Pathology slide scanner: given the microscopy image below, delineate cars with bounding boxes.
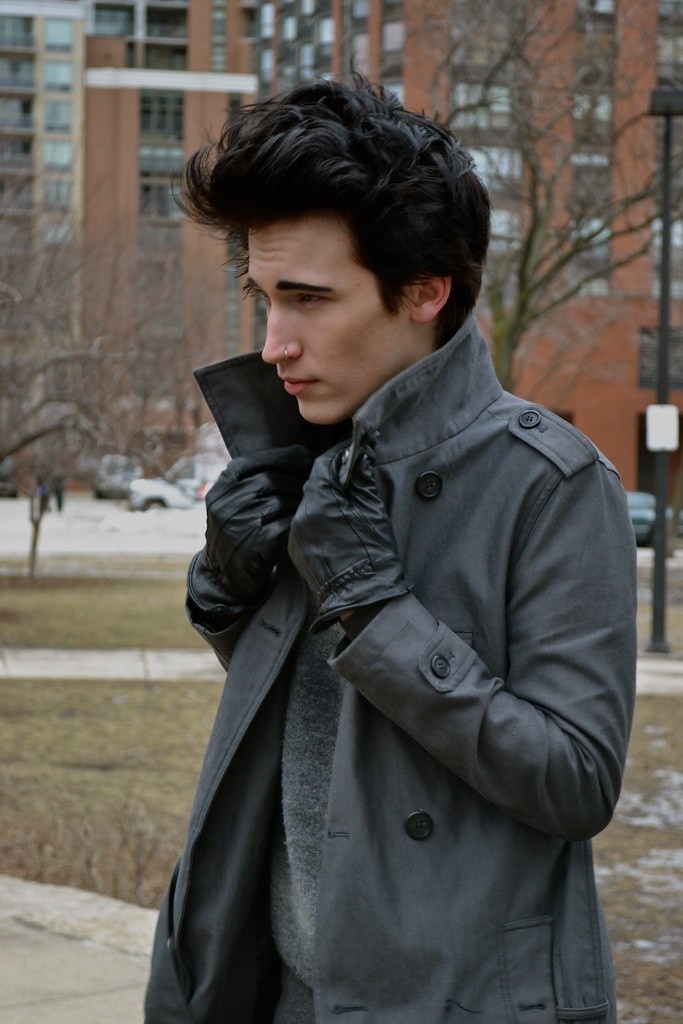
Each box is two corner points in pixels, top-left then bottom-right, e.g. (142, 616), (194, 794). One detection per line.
(623, 491), (673, 545)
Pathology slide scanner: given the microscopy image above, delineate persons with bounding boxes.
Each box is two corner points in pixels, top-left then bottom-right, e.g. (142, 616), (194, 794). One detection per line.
(35, 474), (64, 512)
(142, 54), (638, 1024)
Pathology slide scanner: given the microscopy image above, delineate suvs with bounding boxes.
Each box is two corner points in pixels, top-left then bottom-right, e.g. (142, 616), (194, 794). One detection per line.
(130, 455), (198, 514)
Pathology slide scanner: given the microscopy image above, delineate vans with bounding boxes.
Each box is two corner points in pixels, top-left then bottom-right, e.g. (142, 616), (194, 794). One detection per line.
(95, 455), (144, 499)
(0, 454), (20, 497)
(195, 422), (234, 505)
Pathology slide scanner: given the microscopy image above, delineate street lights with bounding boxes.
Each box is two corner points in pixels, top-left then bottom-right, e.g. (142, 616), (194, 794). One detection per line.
(645, 76), (683, 652)
